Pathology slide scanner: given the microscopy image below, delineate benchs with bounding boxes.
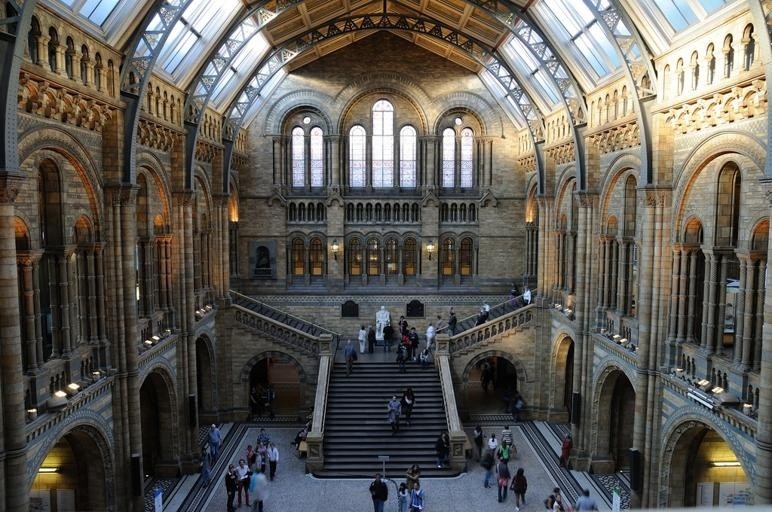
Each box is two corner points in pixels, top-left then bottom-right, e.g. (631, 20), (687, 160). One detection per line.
(463, 431), (473, 459)
(299, 440), (309, 457)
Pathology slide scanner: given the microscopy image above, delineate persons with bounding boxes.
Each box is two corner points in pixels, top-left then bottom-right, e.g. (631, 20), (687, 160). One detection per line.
(435, 431), (450, 469)
(388, 395), (400, 437)
(398, 464), (424, 512)
(249, 387), (262, 419)
(207, 424), (222, 461)
(473, 426), (527, 511)
(478, 360), (524, 422)
(343, 306), (457, 375)
(475, 302), (490, 342)
(400, 387), (415, 426)
(224, 428), (279, 512)
(290, 406), (313, 448)
(369, 474), (388, 512)
(200, 448), (212, 488)
(510, 282), (531, 310)
(544, 487), (597, 512)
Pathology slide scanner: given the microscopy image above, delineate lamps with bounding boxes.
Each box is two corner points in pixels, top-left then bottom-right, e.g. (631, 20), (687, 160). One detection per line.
(707, 461), (741, 467)
(38, 466), (64, 474)
(331, 238), (339, 260)
(554, 303), (753, 417)
(27, 304), (213, 421)
(426, 240), (435, 261)
